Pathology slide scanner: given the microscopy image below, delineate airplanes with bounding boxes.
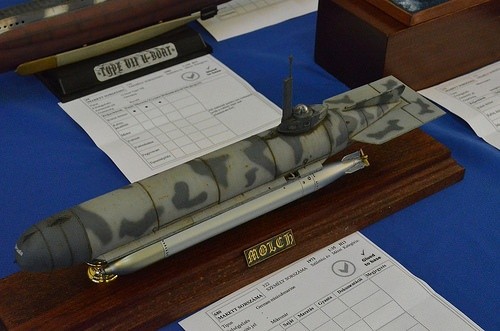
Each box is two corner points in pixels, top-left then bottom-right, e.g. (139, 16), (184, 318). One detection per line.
(0, 0), (232, 76)
(12, 54), (447, 285)
